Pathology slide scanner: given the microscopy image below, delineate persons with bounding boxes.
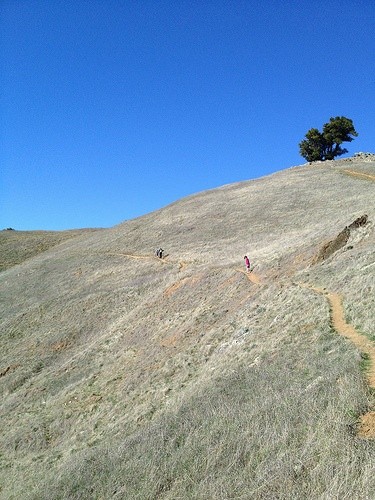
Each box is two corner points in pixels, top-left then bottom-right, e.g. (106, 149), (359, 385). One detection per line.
(156, 250), (159, 257)
(244, 255), (251, 273)
(159, 248), (164, 258)
